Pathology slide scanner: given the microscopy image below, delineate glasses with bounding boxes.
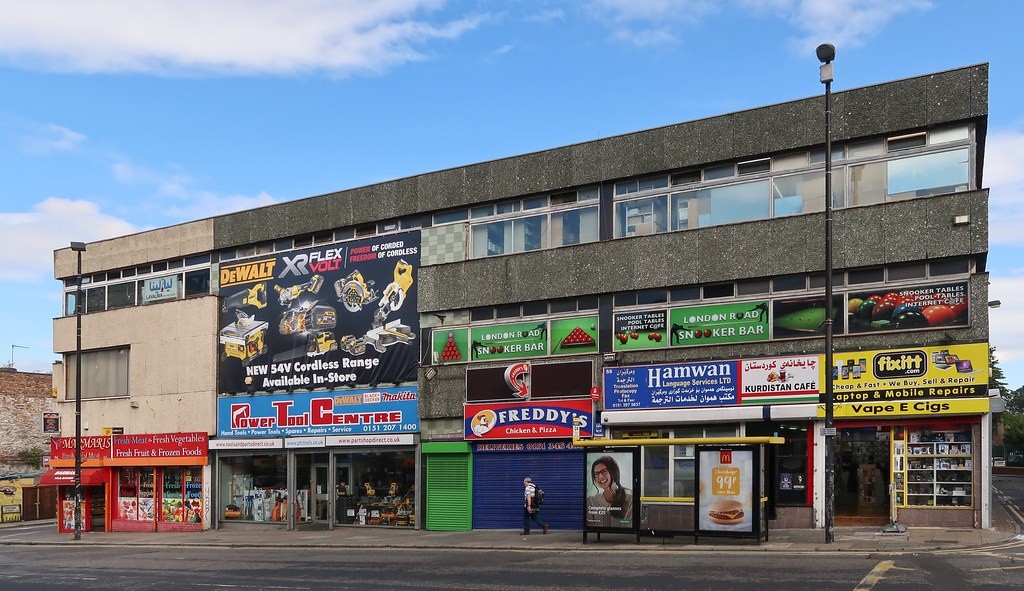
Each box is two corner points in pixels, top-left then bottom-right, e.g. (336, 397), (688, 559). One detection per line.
(593, 468), (609, 478)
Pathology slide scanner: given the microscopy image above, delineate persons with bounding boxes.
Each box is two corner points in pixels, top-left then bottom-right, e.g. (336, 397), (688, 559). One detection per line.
(864, 455), (881, 469)
(844, 457), (859, 510)
(587, 456), (633, 528)
(336, 478), (347, 499)
(520, 477), (549, 535)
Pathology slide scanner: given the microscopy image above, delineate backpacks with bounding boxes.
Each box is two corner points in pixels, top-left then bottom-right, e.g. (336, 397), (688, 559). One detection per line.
(526, 485), (545, 508)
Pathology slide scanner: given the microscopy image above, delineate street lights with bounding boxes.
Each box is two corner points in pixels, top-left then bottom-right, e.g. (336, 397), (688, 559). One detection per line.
(816, 42), (835, 543)
(70, 241), (86, 540)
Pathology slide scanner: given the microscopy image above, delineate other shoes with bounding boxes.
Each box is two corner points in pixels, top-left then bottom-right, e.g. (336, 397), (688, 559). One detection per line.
(520, 531), (530, 535)
(543, 524), (548, 534)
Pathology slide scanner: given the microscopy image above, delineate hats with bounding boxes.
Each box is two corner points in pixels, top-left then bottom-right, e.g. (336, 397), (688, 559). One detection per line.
(524, 478), (533, 483)
